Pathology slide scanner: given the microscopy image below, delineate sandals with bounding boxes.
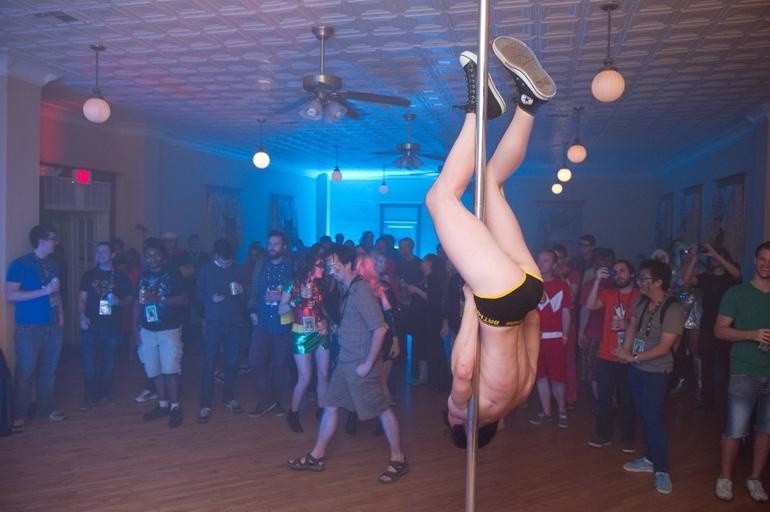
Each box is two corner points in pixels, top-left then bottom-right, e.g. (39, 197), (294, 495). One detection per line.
(567, 400), (577, 411)
(287, 452), (325, 471)
(378, 458), (409, 484)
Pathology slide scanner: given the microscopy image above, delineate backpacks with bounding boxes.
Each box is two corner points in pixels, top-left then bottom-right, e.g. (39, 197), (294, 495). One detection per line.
(658, 296), (693, 395)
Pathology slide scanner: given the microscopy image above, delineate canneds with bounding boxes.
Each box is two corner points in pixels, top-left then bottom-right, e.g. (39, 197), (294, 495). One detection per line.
(52, 277), (60, 283)
(107, 293), (114, 307)
(139, 289), (146, 303)
(230, 281), (238, 296)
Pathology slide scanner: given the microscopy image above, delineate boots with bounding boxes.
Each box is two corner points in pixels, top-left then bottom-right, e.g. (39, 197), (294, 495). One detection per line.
(409, 360), (430, 387)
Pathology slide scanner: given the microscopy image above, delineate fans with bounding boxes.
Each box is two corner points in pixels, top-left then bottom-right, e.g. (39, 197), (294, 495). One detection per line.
(369, 111), (446, 171)
(258, 25), (411, 124)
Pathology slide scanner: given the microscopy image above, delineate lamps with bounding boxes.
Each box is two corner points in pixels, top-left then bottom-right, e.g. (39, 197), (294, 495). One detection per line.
(81, 44), (112, 124)
(393, 153), (423, 171)
(550, 106), (589, 195)
(296, 91), (348, 123)
(251, 118), (387, 195)
(590, 1), (626, 103)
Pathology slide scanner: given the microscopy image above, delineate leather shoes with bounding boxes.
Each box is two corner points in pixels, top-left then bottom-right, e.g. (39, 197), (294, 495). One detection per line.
(8, 416), (25, 433)
(49, 410), (65, 421)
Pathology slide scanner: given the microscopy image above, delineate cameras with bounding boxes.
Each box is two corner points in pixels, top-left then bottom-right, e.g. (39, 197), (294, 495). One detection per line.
(697, 245), (708, 253)
(680, 248), (693, 256)
(607, 267), (616, 277)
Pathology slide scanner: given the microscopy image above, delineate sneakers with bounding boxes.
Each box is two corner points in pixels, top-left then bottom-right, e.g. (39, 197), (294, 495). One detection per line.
(622, 440), (636, 453)
(715, 478), (734, 501)
(458, 51), (507, 121)
(100, 396), (116, 405)
(133, 390), (401, 435)
(655, 471), (673, 494)
(558, 412), (570, 428)
(587, 437), (611, 447)
(746, 478), (768, 502)
(528, 412), (552, 425)
(490, 35), (558, 105)
(622, 456), (654, 473)
(79, 400), (97, 412)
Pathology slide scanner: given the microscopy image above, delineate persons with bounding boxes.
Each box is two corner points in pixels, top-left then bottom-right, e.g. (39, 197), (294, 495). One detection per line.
(424, 34), (557, 451)
(3, 223), (66, 433)
